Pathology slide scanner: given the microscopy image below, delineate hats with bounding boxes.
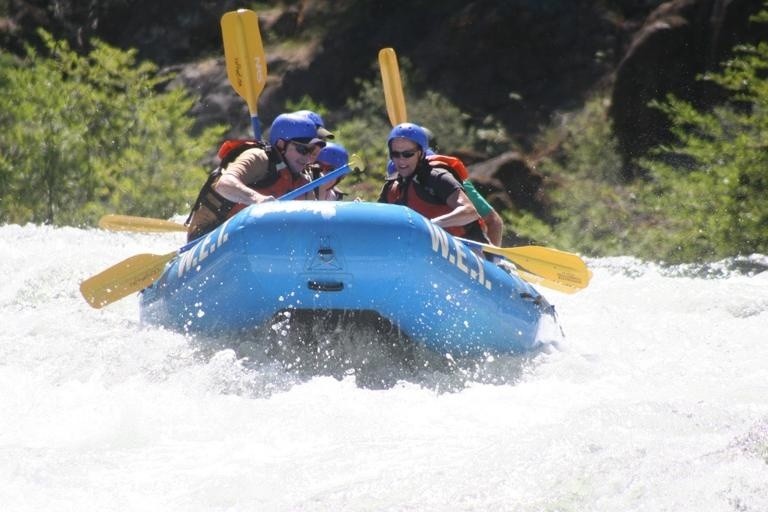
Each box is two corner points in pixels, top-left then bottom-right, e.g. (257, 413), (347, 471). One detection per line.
(290, 138), (326, 148)
(316, 126), (334, 139)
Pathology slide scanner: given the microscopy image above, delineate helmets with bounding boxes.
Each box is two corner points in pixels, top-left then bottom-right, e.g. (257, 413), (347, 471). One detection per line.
(293, 110), (323, 126)
(420, 127), (437, 152)
(388, 123), (426, 153)
(270, 114), (316, 145)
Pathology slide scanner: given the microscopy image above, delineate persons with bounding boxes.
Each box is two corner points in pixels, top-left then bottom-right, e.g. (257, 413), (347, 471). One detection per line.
(183, 111), (337, 247)
(315, 142), (350, 200)
(352, 120), (493, 263)
(276, 110), (334, 199)
(419, 127), (503, 259)
(388, 158), (397, 175)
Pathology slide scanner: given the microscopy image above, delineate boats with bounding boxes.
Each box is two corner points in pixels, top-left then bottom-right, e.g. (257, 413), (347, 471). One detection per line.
(125, 194), (553, 363)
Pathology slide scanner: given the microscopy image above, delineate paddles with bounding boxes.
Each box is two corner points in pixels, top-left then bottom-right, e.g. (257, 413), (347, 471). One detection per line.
(219, 7), (268, 143)
(379, 47), (407, 127)
(450, 235), (593, 295)
(79, 156), (365, 311)
(98, 212), (193, 235)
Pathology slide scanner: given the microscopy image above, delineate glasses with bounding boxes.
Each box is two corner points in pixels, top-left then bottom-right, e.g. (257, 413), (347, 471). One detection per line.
(391, 151), (420, 158)
(293, 143), (318, 155)
(314, 162), (334, 173)
